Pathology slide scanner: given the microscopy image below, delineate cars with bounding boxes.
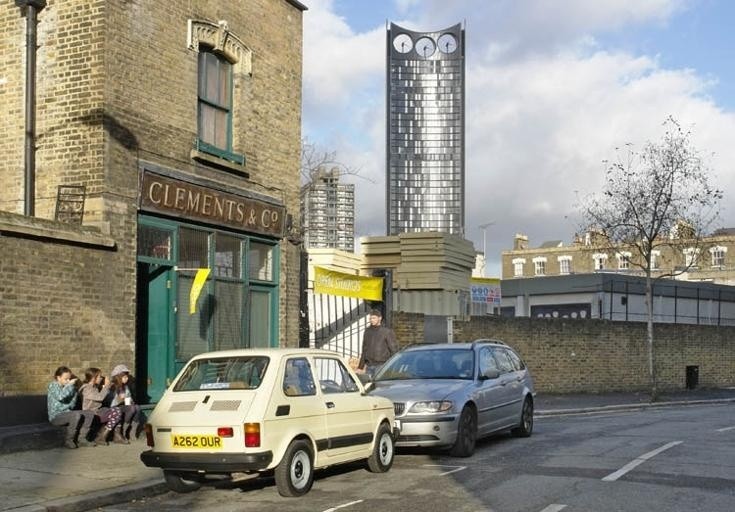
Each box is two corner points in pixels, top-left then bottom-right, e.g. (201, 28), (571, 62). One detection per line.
(356, 336), (538, 462)
(133, 346), (400, 500)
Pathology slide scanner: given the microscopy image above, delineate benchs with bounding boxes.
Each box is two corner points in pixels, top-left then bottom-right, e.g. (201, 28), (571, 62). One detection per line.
(0, 394), (106, 456)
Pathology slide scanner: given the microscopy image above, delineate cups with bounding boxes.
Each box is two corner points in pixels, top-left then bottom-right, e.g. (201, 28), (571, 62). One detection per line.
(123, 397), (131, 405)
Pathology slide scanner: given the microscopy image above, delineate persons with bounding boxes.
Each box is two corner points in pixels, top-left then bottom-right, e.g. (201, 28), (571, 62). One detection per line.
(47, 366), (97, 450)
(357, 310), (398, 382)
(105, 365), (141, 441)
(78, 368), (129, 446)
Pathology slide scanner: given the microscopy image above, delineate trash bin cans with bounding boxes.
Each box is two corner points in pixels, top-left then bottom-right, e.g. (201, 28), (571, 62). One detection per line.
(686, 366), (699, 390)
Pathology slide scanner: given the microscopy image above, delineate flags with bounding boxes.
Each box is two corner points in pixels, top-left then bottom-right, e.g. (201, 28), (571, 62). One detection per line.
(190, 268), (211, 313)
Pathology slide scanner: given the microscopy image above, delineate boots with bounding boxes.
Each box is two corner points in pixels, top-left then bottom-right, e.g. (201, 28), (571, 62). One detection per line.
(113, 424), (127, 444)
(65, 427), (77, 448)
(130, 421), (140, 441)
(78, 426), (96, 447)
(96, 426), (111, 446)
(122, 421), (130, 442)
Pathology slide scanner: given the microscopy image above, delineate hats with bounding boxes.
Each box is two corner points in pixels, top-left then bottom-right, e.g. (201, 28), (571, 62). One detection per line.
(111, 365), (130, 377)
